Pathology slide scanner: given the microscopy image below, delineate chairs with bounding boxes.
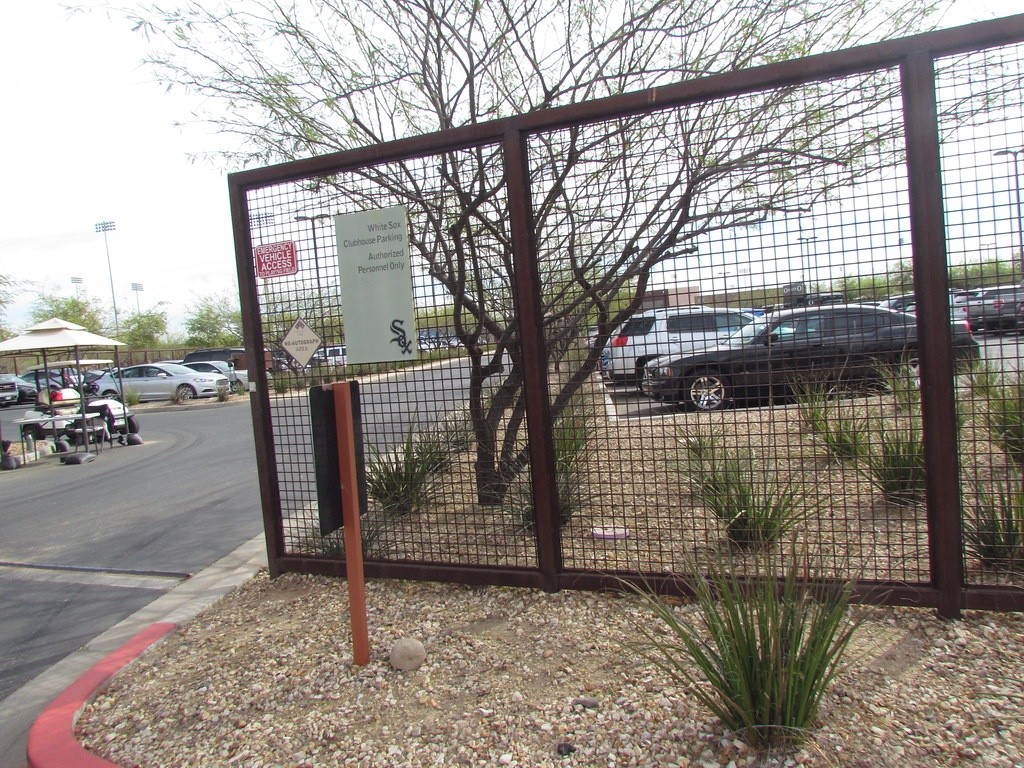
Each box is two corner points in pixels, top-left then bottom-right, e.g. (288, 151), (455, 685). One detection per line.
(814, 320), (824, 331)
(38, 389), (50, 405)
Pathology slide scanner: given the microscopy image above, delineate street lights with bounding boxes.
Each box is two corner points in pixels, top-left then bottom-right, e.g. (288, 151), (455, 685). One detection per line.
(295, 214), (331, 363)
(797, 237), (819, 306)
(71, 277), (83, 300)
(95, 220), (118, 335)
(718, 272), (731, 307)
(995, 149), (1023, 279)
(132, 283), (144, 314)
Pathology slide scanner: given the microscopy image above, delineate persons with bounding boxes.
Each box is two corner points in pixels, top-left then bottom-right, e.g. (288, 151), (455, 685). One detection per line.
(49, 384), (63, 416)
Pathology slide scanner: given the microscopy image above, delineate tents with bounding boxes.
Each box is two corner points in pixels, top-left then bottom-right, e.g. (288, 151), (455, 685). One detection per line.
(0, 318), (129, 453)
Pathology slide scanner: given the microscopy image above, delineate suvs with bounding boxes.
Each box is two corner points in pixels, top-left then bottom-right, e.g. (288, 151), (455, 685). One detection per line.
(605, 307), (816, 397)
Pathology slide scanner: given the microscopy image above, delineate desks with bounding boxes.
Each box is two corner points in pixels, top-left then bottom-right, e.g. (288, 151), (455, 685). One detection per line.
(13, 413), (100, 465)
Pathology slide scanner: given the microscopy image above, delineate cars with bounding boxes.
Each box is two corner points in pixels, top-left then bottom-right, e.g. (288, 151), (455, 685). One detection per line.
(642, 304), (981, 412)
(16, 376), (48, 405)
(18, 367), (87, 399)
(81, 370), (105, 392)
(314, 335), (465, 365)
(183, 361), (274, 394)
(864, 285), (1024, 338)
(0, 374), (19, 408)
(88, 364), (232, 404)
